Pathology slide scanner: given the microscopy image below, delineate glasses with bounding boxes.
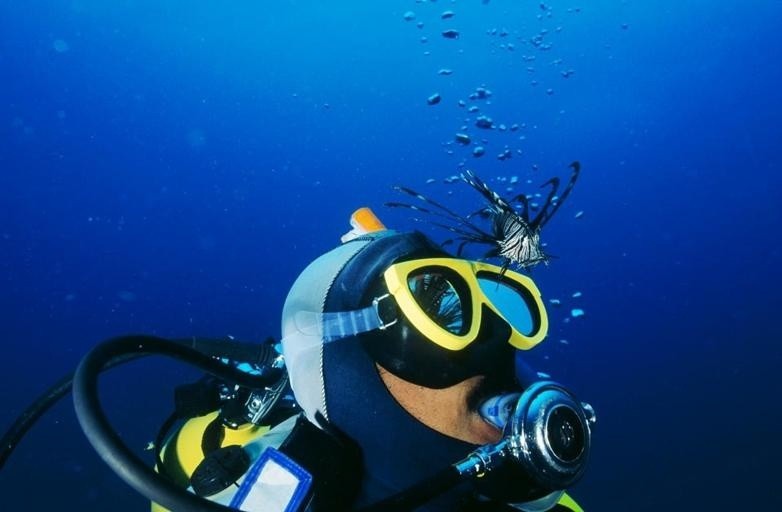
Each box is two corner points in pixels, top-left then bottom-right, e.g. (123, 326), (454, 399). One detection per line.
(373, 251), (553, 356)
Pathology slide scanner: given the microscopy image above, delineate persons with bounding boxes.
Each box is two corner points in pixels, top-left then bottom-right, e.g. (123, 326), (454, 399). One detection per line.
(151, 204), (581, 512)
